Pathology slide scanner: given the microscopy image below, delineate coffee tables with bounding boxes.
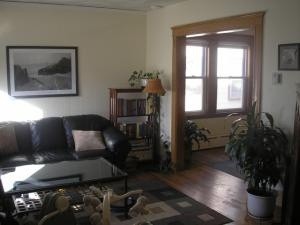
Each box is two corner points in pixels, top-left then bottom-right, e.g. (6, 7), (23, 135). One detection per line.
(0, 155), (128, 216)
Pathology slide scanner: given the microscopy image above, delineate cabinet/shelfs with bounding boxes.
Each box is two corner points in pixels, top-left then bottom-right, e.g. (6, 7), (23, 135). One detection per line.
(108, 87), (161, 168)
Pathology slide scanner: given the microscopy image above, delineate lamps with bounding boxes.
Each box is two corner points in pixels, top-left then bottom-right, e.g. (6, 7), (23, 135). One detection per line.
(144, 78), (164, 168)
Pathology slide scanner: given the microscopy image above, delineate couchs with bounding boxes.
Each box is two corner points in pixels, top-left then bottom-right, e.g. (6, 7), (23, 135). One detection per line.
(0, 114), (132, 193)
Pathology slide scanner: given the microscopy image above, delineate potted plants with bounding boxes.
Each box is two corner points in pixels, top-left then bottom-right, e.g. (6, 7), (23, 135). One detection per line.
(184, 120), (213, 159)
(128, 69), (174, 171)
(224, 101), (290, 219)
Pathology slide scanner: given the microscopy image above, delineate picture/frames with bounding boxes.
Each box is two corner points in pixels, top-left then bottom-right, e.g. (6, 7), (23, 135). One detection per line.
(278, 43), (300, 71)
(5, 45), (80, 98)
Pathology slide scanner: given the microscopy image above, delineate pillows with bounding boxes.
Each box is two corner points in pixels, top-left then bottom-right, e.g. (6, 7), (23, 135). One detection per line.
(71, 130), (106, 152)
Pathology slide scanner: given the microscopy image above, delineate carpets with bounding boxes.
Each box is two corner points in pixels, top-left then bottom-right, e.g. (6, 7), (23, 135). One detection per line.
(0, 165), (234, 225)
(193, 145), (249, 181)
